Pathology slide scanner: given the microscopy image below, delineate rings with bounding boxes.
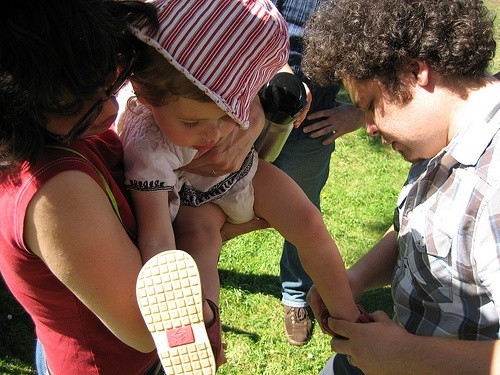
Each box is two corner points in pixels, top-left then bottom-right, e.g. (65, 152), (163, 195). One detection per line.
(212, 170), (218, 175)
(346, 356), (353, 368)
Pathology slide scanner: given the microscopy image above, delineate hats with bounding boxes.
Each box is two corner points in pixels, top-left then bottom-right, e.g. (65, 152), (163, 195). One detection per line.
(125, 0), (291, 129)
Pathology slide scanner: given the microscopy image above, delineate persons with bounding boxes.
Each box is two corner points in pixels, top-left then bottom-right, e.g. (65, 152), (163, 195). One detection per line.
(114, 1), (376, 375)
(0, 0), (270, 375)
(302, 0), (500, 375)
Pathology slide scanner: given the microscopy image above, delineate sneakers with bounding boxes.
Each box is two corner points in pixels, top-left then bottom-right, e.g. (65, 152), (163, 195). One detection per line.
(284, 303), (313, 345)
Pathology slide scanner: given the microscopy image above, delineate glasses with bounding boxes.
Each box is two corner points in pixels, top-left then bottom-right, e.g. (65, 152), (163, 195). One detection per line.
(32, 45), (139, 145)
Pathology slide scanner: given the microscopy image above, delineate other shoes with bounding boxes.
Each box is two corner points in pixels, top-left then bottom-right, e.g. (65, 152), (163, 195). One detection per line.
(321, 307), (377, 340)
(135, 249), (218, 375)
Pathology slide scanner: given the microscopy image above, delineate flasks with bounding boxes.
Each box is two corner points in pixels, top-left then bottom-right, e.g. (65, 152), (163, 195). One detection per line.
(255, 72), (307, 163)
